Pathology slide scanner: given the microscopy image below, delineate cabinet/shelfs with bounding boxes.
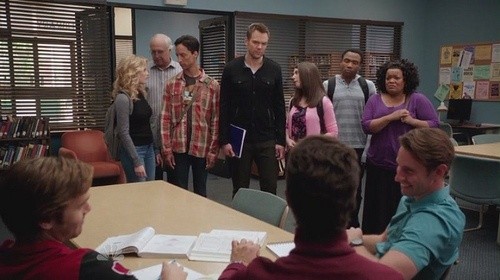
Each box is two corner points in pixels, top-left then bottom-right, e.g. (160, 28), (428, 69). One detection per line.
(368, 51), (392, 77)
(288, 53), (332, 89)
(0, 122), (52, 171)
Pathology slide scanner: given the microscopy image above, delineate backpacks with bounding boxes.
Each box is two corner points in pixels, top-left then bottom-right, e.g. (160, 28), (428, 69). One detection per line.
(104, 89), (131, 161)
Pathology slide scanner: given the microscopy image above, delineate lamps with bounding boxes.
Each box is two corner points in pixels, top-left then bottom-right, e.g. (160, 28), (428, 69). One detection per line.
(436, 101), (448, 121)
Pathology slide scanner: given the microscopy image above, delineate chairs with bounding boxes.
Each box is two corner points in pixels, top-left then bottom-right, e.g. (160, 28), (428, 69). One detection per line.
(440, 122), (500, 232)
(230, 187), (290, 229)
(59, 130), (127, 184)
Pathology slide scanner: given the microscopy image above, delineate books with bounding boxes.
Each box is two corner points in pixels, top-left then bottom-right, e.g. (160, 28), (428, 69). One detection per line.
(95, 227), (198, 259)
(0, 144), (49, 169)
(265, 241), (296, 257)
(189, 228), (267, 263)
(0, 117), (48, 140)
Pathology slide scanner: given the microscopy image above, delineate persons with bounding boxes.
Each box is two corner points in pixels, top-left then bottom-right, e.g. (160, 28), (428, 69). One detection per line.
(160, 35), (221, 198)
(218, 22), (286, 199)
(217, 135), (406, 280)
(111, 53), (163, 183)
(347, 128), (466, 280)
(361, 59), (439, 236)
(142, 34), (183, 180)
(285, 62), (338, 150)
(323, 48), (378, 229)
(0, 156), (188, 280)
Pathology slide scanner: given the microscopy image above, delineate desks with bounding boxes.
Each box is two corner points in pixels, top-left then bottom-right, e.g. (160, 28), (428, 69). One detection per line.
(454, 142), (500, 243)
(452, 124), (500, 145)
(68, 180), (295, 280)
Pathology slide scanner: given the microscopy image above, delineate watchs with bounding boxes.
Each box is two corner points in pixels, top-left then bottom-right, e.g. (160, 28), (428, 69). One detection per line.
(349, 240), (363, 247)
